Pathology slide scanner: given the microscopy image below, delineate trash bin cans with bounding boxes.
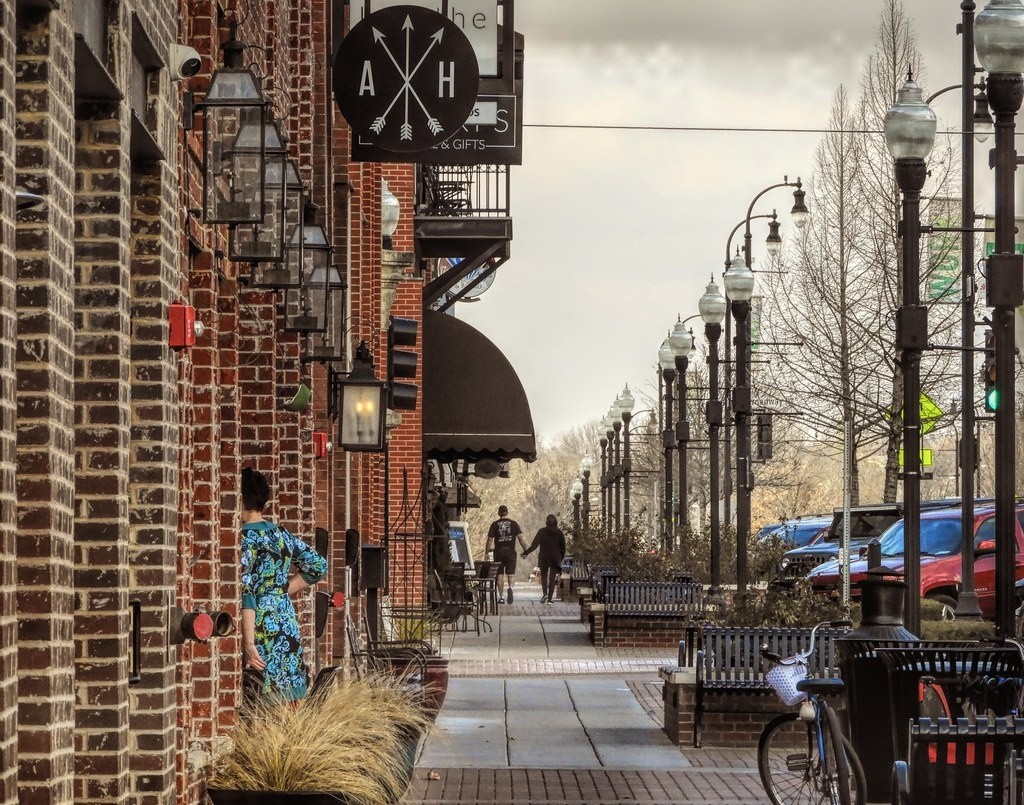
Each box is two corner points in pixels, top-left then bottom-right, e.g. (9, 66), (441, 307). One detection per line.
(833, 639), (979, 805)
(874, 648), (1024, 805)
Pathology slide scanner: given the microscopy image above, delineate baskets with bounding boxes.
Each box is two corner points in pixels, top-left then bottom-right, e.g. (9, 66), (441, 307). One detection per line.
(765, 656), (814, 706)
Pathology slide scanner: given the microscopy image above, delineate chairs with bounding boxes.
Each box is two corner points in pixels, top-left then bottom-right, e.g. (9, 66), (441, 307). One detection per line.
(433, 560), (501, 633)
(361, 610), (438, 655)
(342, 613), (433, 734)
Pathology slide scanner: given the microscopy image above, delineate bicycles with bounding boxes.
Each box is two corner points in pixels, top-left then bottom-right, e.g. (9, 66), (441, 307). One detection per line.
(758, 620), (868, 805)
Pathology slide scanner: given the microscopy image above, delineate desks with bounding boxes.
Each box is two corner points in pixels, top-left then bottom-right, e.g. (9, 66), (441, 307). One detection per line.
(444, 577), (494, 637)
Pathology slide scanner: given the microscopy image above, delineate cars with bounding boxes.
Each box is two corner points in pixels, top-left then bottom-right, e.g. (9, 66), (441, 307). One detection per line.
(748, 514), (834, 548)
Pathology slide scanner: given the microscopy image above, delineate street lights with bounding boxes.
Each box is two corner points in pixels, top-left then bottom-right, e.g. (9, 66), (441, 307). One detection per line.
(883, 64), (938, 645)
(604, 411), (615, 540)
(610, 395), (622, 534)
(725, 244), (754, 614)
(597, 414), (609, 540)
(570, 450), (593, 537)
(618, 382), (635, 542)
(699, 272), (726, 607)
(974, 0), (1023, 643)
(659, 329), (676, 552)
(670, 313), (692, 584)
(745, 175), (809, 537)
(723, 209), (782, 529)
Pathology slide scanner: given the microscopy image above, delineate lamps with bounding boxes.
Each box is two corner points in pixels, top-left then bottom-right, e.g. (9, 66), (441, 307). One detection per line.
(182, 0), (393, 455)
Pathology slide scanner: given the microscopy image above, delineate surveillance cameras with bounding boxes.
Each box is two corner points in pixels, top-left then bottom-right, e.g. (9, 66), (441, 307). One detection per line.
(169, 43), (201, 81)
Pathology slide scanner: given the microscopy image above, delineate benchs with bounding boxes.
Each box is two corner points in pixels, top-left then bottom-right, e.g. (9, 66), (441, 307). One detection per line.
(563, 556), (1024, 805)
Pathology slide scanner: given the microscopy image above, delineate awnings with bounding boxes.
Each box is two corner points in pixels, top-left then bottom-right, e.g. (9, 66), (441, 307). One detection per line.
(422, 308), (538, 464)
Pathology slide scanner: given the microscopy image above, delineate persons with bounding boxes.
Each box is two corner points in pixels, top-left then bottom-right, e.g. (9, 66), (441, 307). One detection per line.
(240, 467), (328, 712)
(521, 514), (566, 604)
(484, 506), (526, 603)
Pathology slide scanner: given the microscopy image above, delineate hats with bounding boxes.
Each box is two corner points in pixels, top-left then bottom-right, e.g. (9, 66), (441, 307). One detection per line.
(498, 506), (508, 516)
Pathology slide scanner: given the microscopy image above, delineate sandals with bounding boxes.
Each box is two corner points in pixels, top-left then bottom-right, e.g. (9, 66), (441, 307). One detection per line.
(507, 587), (514, 604)
(498, 597), (505, 603)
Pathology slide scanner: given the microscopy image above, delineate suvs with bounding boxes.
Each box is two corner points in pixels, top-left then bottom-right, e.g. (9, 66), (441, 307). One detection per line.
(779, 498), (995, 581)
(804, 496), (1024, 622)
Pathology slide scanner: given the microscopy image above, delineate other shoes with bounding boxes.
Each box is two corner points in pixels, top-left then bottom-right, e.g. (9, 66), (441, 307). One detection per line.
(548, 601), (553, 604)
(540, 594), (548, 603)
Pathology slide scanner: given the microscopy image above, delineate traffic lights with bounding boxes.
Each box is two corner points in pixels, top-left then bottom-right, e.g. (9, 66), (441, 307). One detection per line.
(387, 315), (417, 411)
(985, 329), (998, 413)
